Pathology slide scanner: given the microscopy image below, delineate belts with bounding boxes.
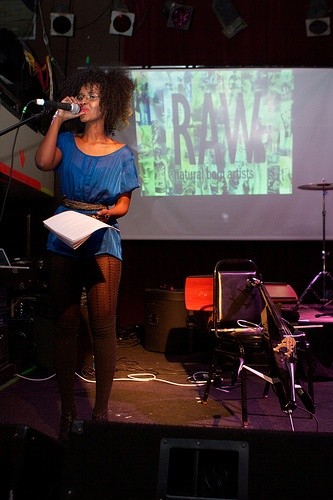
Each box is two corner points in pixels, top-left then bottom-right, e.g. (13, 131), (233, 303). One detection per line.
(59, 199), (108, 211)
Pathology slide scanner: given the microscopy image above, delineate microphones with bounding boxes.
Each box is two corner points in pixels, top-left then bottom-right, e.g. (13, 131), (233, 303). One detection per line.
(35, 98), (81, 114)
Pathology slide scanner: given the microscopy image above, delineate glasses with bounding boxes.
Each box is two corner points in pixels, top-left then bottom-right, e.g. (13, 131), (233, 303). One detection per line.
(74, 94), (100, 102)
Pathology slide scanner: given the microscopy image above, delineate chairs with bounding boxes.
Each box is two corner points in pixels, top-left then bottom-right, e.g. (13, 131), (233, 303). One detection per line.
(204, 256), (283, 427)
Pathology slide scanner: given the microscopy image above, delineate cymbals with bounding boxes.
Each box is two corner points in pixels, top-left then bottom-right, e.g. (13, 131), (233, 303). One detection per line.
(297, 183), (333, 191)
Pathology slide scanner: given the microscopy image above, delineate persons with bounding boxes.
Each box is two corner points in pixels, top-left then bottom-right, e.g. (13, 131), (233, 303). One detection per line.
(33, 66), (139, 447)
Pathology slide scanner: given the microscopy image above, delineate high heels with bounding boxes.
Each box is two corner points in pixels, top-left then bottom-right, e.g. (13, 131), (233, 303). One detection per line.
(57, 412), (74, 439)
